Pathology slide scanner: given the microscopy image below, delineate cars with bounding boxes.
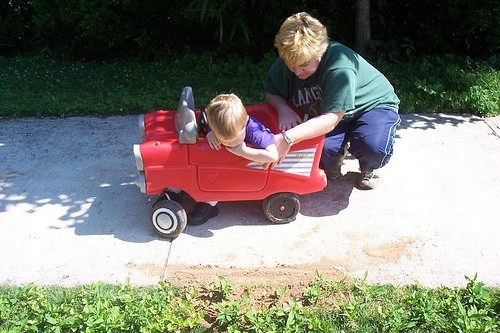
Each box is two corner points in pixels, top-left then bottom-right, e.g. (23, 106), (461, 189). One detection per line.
(132, 86), (328, 240)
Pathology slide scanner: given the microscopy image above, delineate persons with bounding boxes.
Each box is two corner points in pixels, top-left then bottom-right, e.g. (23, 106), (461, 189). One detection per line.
(264, 12), (401, 188)
(150, 94), (279, 226)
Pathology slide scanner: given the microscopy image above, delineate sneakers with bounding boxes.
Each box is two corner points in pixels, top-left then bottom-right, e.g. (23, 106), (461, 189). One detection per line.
(358, 167), (381, 189)
(153, 188), (184, 209)
(187, 201), (219, 225)
(326, 142), (349, 181)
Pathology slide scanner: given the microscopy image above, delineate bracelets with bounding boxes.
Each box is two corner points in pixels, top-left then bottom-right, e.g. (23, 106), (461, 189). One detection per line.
(281, 129), (293, 146)
